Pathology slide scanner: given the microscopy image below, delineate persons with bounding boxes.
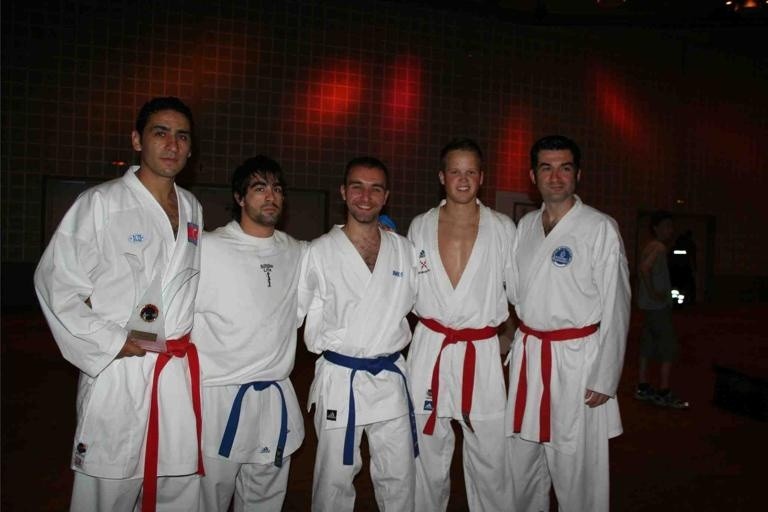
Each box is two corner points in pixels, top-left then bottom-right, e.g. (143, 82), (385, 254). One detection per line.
(506, 136), (631, 512)
(187, 151), (397, 509)
(293, 151), (420, 512)
(29, 90), (206, 512)
(401, 137), (519, 512)
(636, 217), (691, 409)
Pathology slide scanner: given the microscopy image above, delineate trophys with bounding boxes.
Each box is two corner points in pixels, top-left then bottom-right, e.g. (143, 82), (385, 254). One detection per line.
(115, 246), (201, 355)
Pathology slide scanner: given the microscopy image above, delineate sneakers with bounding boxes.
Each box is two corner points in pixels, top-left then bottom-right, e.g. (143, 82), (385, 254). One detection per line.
(633, 390), (682, 407)
